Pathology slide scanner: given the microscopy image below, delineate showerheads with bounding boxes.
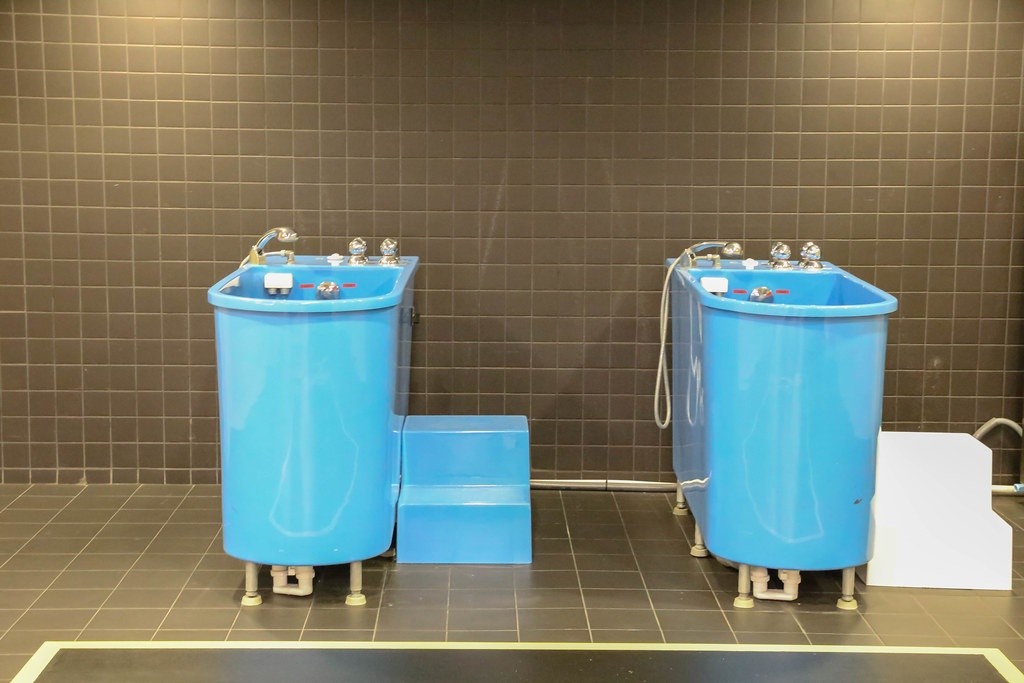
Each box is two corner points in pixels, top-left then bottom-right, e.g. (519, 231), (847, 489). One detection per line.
(687, 241), (744, 258)
(255, 226), (299, 254)
